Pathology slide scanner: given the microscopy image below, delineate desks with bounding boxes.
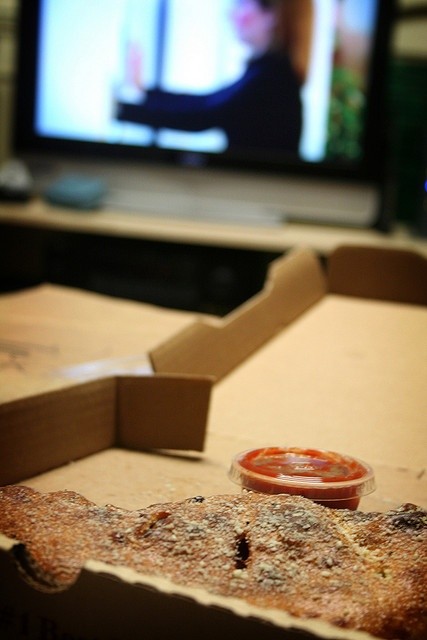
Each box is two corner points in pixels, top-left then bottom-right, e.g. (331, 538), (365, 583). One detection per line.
(0, 196), (426, 313)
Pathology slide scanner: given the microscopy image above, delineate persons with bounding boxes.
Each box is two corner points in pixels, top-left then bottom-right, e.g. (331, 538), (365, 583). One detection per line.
(110, 1), (304, 161)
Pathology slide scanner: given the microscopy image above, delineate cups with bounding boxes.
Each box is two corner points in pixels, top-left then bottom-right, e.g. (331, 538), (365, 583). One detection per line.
(226, 446), (377, 511)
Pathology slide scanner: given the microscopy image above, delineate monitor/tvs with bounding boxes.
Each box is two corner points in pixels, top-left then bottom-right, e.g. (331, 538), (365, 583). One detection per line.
(10, 0), (397, 229)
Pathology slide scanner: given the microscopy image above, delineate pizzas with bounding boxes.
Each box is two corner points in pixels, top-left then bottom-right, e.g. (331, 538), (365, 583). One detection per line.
(0, 485), (427, 639)
